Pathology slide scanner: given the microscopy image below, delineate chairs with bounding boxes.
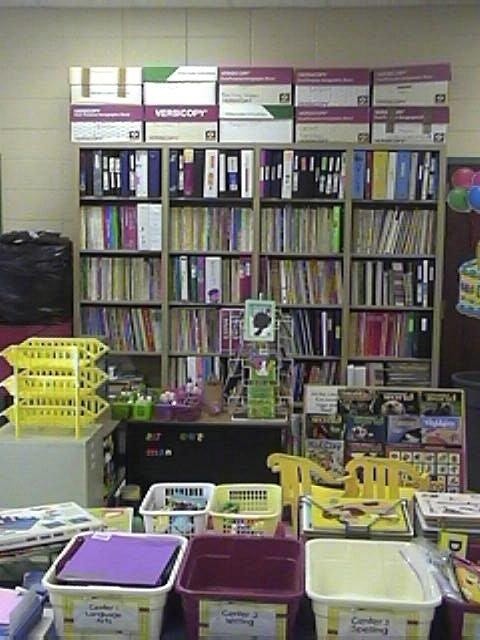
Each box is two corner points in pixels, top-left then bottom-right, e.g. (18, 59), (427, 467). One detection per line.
(265, 453), (429, 500)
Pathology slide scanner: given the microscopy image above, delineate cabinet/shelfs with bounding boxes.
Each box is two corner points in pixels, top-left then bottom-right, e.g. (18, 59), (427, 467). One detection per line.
(1, 421), (106, 512)
(73, 142), (447, 414)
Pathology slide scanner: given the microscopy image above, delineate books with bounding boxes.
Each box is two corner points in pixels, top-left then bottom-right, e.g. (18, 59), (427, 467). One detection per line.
(297, 491), (480, 561)
(1, 500), (136, 561)
(74, 148), (163, 417)
(353, 151), (441, 388)
(260, 150), (344, 405)
(169, 148), (252, 408)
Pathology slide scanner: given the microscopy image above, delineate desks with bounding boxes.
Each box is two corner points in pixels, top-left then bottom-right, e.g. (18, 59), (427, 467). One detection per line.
(125, 404), (290, 500)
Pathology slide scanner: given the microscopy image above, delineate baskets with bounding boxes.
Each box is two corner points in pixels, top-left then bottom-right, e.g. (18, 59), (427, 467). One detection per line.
(205, 480), (286, 539)
(138, 482), (214, 536)
